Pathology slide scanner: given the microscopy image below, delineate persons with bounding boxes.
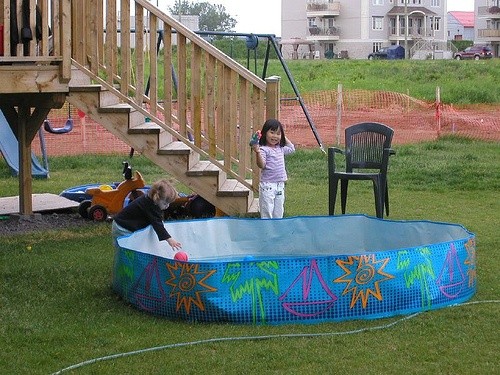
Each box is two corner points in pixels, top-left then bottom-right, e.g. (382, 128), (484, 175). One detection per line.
(251, 118), (297, 219)
(111, 177), (185, 251)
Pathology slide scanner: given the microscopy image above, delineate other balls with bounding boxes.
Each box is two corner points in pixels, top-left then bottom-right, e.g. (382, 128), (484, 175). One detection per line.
(174, 253), (188, 262)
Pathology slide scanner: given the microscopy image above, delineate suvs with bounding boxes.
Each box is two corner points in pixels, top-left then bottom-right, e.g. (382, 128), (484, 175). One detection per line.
(368, 47), (389, 59)
(452, 45), (493, 60)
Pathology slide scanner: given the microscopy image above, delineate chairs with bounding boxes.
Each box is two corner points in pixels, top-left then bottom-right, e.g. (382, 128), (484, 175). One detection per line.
(327, 122), (397, 221)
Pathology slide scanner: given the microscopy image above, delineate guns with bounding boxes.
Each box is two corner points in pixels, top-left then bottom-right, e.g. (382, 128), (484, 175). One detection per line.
(249, 129), (261, 146)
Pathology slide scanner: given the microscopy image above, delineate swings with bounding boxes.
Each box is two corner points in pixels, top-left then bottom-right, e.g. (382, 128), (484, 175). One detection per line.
(44, 102), (73, 134)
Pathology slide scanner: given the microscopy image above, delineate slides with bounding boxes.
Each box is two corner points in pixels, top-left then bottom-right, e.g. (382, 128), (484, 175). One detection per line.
(1, 110), (48, 177)
